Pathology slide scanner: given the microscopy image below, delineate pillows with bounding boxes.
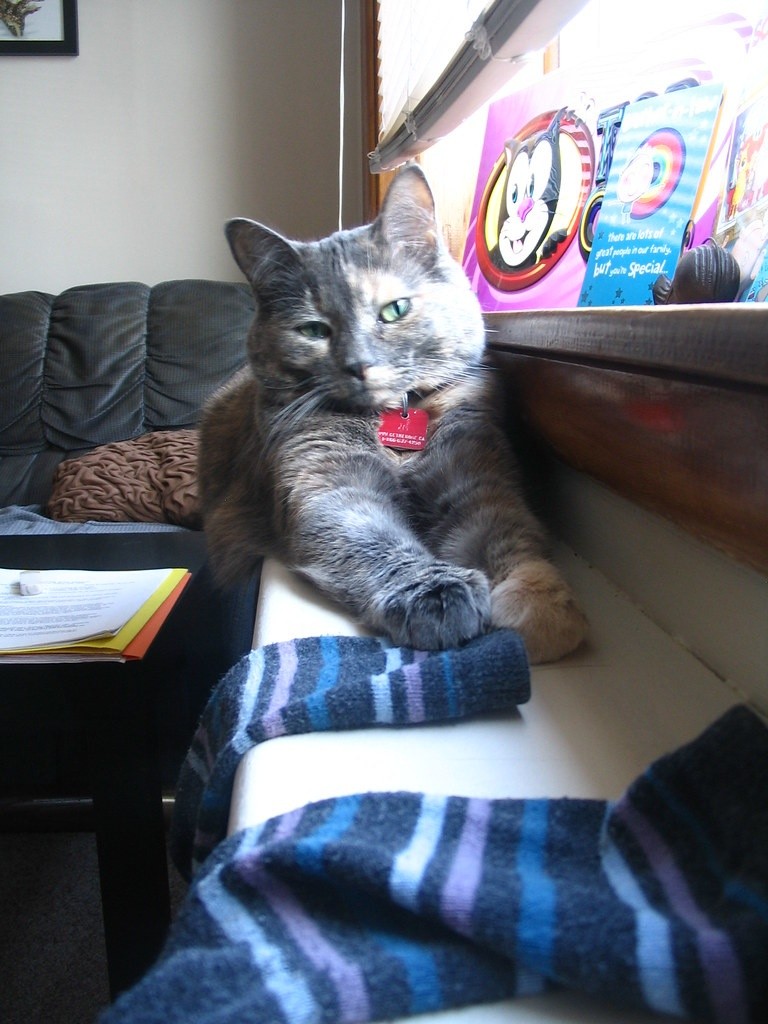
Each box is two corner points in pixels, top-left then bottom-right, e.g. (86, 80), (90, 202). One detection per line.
(47, 429), (200, 527)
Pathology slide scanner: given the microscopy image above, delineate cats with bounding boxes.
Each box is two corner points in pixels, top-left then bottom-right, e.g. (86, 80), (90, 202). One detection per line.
(198, 163), (589, 670)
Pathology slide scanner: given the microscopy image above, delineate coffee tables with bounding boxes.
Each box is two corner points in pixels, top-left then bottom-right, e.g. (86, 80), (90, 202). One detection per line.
(0, 533), (213, 1005)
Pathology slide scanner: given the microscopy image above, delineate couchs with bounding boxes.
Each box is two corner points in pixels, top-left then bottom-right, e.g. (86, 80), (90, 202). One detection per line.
(0, 279), (255, 532)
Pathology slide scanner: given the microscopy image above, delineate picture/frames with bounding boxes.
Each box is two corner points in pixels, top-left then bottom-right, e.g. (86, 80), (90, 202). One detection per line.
(0, 0), (80, 56)
